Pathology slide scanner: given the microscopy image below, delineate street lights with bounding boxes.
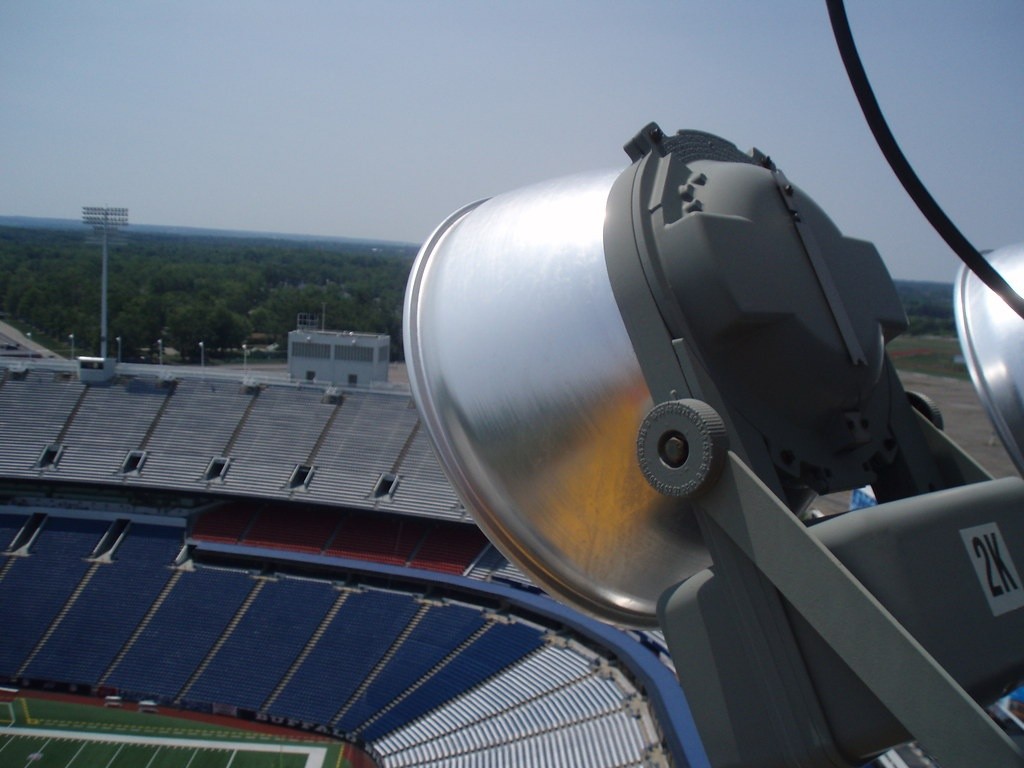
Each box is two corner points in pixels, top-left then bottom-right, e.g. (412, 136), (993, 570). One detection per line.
(116, 337), (122, 363)
(68, 334), (75, 360)
(157, 338), (164, 363)
(199, 342), (205, 368)
(242, 344), (247, 368)
(27, 331), (33, 361)
